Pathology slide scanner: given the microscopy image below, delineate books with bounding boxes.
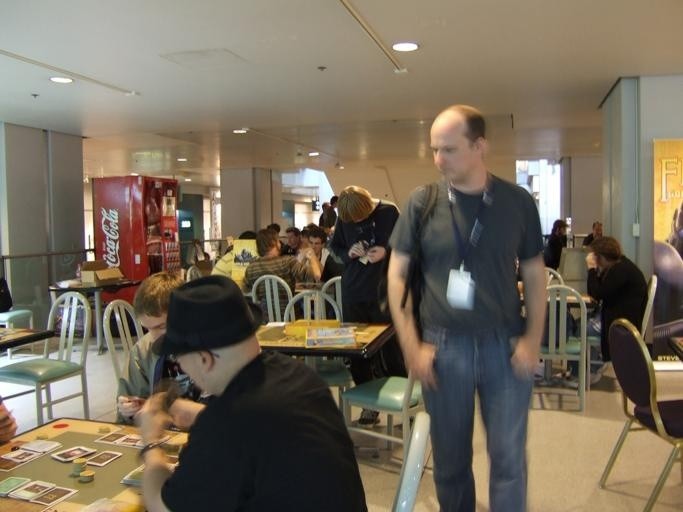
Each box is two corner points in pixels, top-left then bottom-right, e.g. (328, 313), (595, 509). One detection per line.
(305, 326), (354, 339)
(305, 337), (357, 348)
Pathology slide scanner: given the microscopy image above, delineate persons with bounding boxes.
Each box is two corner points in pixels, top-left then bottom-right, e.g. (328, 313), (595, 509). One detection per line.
(384, 102), (550, 512)
(131, 273), (371, 512)
(113, 270), (215, 433)
(518, 217), (647, 389)
(193, 196), (336, 326)
(0, 394), (19, 445)
(326, 185), (403, 431)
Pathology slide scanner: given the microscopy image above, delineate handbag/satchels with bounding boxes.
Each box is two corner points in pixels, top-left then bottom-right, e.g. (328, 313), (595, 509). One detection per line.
(378, 183), (437, 322)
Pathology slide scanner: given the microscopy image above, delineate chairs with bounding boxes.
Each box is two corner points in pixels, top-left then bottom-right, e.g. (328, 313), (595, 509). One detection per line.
(322, 275), (344, 322)
(599, 315), (681, 512)
(283, 288), (342, 321)
(2, 248), (147, 427)
(517, 248), (659, 412)
(338, 361), (429, 470)
(250, 275), (297, 322)
(186, 265), (204, 284)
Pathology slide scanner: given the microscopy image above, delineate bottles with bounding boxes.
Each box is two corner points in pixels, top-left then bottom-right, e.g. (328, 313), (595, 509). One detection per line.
(146, 187), (163, 274)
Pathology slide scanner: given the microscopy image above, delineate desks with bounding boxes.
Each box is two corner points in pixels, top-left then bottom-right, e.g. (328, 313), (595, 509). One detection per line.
(254, 321), (395, 425)
(0, 415), (190, 511)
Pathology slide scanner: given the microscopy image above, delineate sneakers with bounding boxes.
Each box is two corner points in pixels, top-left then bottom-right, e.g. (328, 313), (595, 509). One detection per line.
(590, 362), (610, 384)
(357, 409), (377, 428)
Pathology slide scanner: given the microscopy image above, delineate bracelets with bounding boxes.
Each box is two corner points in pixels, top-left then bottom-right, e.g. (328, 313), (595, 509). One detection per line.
(140, 442), (162, 457)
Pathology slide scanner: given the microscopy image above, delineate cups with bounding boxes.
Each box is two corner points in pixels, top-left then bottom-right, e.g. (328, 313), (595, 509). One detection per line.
(80, 471), (94, 483)
(72, 458), (88, 476)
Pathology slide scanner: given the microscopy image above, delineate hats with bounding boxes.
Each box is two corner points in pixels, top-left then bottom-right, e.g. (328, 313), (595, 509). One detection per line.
(152, 275), (260, 354)
